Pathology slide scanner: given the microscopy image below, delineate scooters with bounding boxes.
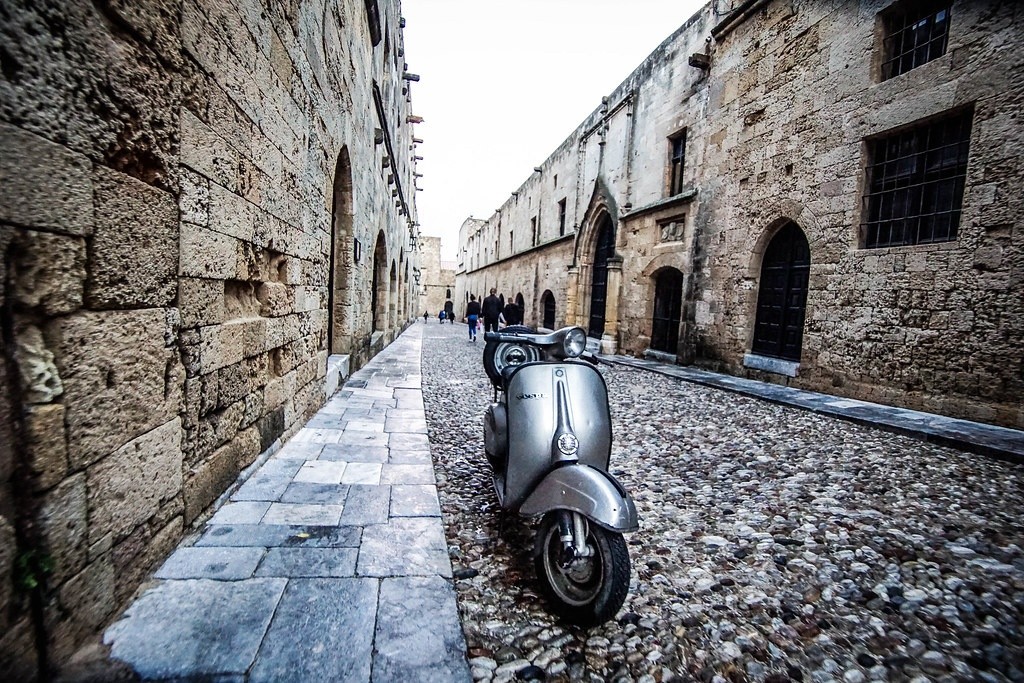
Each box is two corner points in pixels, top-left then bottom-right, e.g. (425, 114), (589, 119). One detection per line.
(481, 322), (641, 628)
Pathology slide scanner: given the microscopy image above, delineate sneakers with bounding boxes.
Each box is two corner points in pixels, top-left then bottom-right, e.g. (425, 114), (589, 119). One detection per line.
(473, 335), (476, 341)
(469, 339), (473, 343)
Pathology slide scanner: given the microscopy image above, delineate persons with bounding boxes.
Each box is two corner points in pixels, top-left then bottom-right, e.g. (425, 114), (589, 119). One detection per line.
(481, 288), (501, 341)
(438, 310), (446, 324)
(449, 312), (455, 324)
(502, 298), (521, 327)
(465, 293), (480, 343)
(423, 311), (429, 324)
